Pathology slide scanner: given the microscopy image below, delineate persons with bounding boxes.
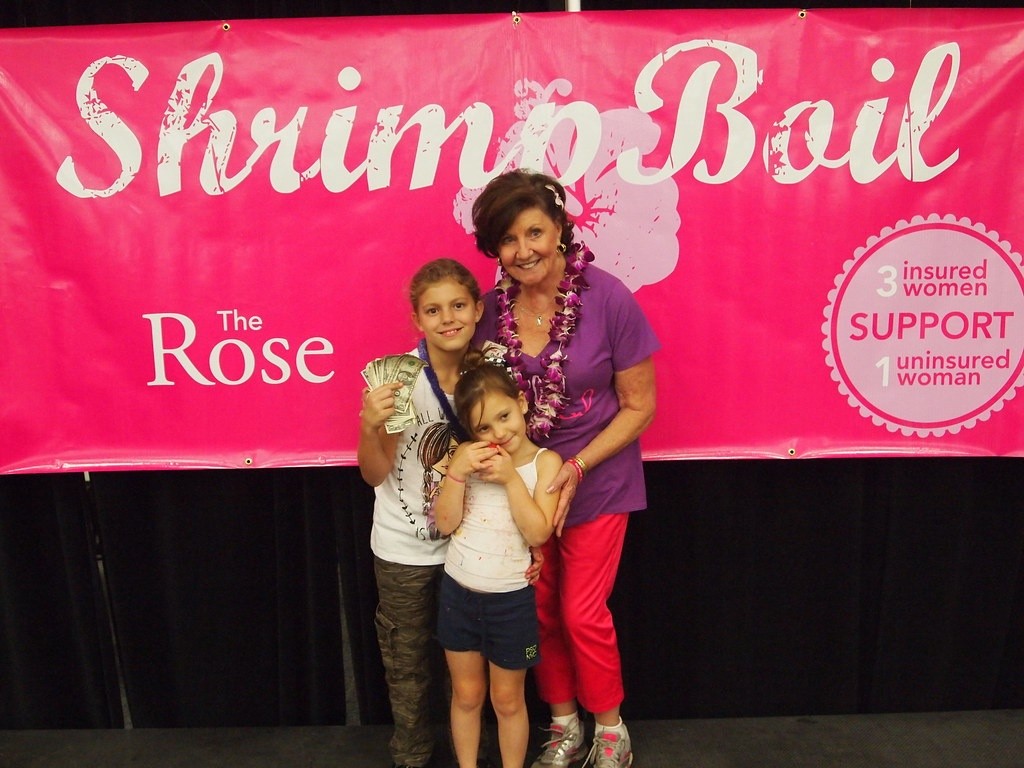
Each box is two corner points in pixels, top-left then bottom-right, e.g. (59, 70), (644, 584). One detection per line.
(358, 168), (662, 768)
(433, 344), (563, 768)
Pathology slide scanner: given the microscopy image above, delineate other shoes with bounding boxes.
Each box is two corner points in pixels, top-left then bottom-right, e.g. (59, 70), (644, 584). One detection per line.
(478, 756), (496, 768)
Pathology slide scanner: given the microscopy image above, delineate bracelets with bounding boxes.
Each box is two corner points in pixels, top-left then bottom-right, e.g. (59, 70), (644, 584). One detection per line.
(446, 473), (466, 484)
(567, 456), (588, 491)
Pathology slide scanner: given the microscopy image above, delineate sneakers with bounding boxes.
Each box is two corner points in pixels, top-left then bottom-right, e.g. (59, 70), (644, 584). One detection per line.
(531, 716), (588, 768)
(582, 724), (633, 768)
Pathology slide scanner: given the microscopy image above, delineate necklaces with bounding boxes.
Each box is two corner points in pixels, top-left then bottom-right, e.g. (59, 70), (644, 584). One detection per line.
(513, 293), (557, 327)
(495, 239), (596, 444)
